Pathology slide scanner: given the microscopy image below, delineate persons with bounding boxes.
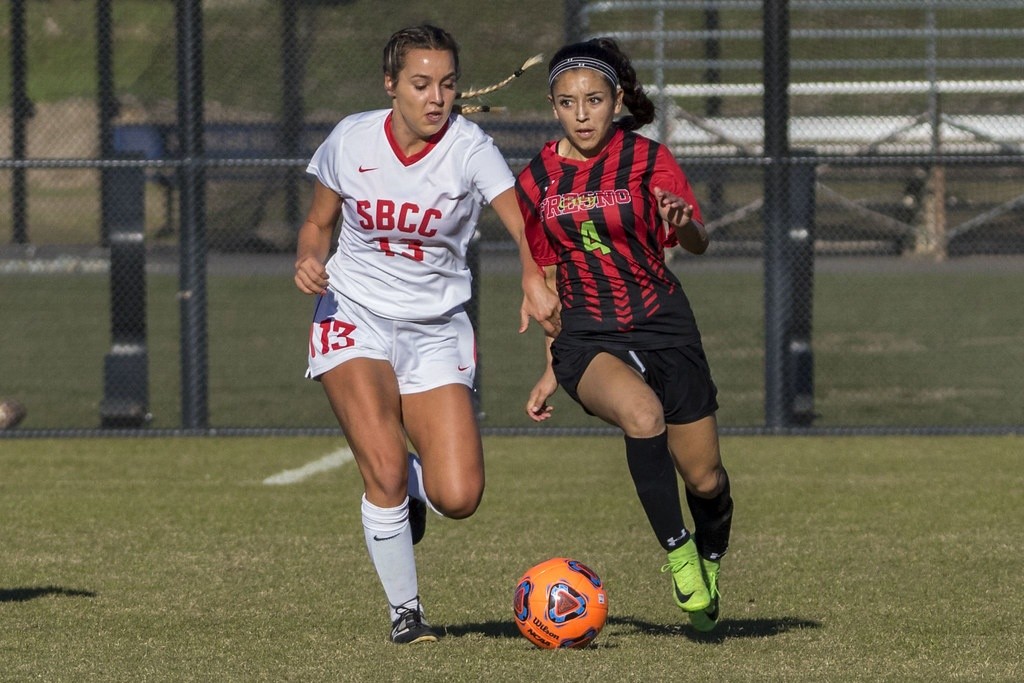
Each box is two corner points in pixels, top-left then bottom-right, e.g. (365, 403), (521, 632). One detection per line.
(523, 34), (735, 635)
(291, 24), (565, 649)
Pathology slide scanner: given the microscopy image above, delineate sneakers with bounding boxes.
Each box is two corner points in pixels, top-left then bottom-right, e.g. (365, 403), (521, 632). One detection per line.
(405, 452), (427, 543)
(691, 556), (719, 635)
(659, 529), (709, 612)
(386, 600), (442, 646)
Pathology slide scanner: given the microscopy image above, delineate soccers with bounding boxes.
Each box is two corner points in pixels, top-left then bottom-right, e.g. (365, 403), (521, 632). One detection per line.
(510, 555), (614, 652)
